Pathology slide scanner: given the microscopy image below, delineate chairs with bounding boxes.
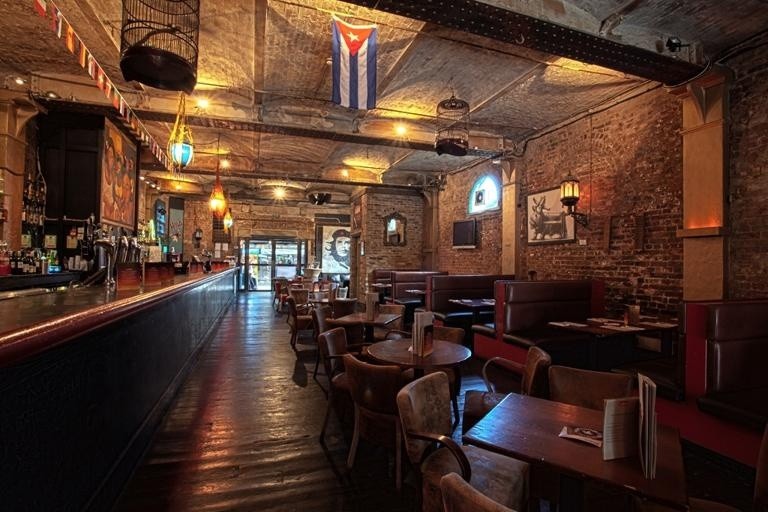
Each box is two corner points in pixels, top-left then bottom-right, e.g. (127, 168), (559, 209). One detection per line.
(682, 422), (768, 511)
(271, 273), (634, 512)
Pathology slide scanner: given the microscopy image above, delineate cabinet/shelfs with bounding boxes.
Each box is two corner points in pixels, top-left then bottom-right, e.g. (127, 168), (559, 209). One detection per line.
(40, 107), (139, 235)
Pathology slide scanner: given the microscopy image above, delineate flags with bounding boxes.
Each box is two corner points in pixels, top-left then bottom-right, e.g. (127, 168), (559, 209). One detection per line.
(331, 15), (378, 110)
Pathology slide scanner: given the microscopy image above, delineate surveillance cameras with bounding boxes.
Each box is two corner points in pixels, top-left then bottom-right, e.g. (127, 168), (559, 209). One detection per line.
(687, 43), (704, 66)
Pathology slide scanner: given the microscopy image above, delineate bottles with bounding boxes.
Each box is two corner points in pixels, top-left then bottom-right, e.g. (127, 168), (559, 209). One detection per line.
(9, 247), (57, 275)
(63, 256), (67, 268)
(23, 173), (45, 248)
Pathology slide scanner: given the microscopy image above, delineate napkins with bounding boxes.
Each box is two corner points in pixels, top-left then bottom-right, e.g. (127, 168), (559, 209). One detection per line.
(640, 321), (677, 328)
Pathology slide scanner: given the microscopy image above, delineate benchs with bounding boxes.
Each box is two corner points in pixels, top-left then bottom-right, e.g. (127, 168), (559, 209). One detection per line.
(694, 302), (768, 434)
(607, 354), (686, 403)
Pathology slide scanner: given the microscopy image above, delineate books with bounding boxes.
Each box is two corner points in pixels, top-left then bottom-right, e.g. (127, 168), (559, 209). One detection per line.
(601, 373), (659, 480)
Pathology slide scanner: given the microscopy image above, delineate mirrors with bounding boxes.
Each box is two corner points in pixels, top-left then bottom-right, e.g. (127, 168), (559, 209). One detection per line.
(383, 211), (408, 248)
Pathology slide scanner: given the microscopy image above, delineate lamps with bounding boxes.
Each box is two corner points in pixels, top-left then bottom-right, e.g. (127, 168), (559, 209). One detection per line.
(193, 226), (202, 248)
(221, 200), (234, 229)
(558, 170), (590, 229)
(162, 85), (195, 173)
(206, 132), (227, 216)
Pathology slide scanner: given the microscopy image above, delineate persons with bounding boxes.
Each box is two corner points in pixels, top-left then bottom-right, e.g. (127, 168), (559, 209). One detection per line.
(322, 230), (352, 273)
(104, 137), (134, 224)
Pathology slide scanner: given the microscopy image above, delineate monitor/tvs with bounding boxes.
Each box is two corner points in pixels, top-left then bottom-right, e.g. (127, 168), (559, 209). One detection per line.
(451, 217), (476, 249)
